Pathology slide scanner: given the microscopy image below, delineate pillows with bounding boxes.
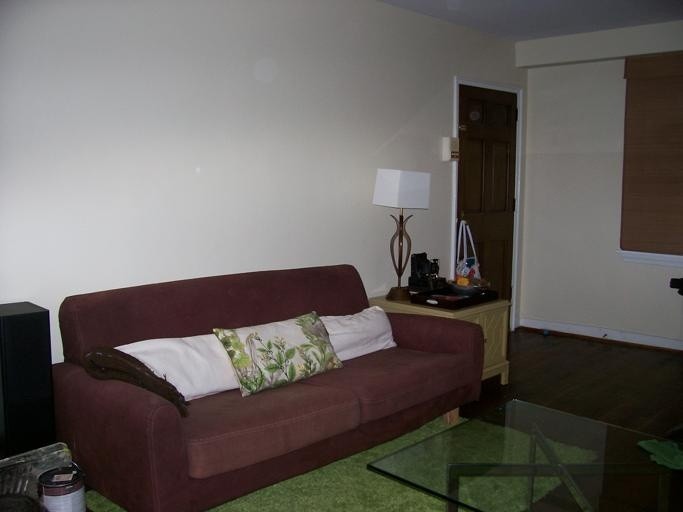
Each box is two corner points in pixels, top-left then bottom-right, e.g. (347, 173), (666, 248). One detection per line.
(320, 306), (397, 361)
(111, 333), (239, 401)
(212, 311), (345, 399)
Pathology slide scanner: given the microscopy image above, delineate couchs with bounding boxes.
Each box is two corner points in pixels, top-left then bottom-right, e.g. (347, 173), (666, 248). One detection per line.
(54, 264), (485, 512)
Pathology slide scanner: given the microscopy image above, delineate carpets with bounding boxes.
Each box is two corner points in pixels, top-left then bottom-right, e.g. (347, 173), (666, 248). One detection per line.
(86, 416), (599, 511)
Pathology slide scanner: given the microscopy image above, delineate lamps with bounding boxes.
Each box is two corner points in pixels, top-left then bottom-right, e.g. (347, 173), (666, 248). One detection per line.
(372, 168), (430, 302)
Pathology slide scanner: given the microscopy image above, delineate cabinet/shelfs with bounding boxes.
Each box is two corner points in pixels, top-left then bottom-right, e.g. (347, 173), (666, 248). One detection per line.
(368, 287), (512, 387)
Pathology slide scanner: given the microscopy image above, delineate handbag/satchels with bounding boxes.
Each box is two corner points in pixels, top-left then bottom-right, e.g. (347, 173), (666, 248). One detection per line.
(455, 256), (482, 288)
